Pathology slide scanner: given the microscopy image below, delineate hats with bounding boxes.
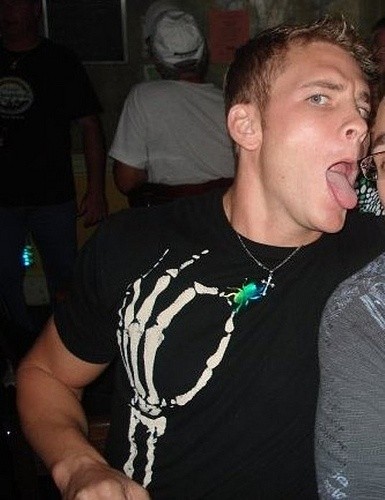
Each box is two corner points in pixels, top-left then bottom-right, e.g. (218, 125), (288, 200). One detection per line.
(149, 9), (204, 70)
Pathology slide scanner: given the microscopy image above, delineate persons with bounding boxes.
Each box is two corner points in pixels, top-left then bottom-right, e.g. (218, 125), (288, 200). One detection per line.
(0, 0), (107, 388)
(107, 0), (238, 206)
(15, 15), (385, 500)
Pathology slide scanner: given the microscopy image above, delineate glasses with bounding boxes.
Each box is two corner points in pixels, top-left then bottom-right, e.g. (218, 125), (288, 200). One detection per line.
(357, 151), (384, 181)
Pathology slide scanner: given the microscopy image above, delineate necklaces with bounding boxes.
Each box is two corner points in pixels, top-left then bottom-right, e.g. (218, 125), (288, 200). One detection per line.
(232, 226), (305, 295)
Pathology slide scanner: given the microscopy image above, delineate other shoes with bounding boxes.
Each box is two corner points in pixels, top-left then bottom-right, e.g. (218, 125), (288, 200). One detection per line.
(4, 358), (16, 389)
